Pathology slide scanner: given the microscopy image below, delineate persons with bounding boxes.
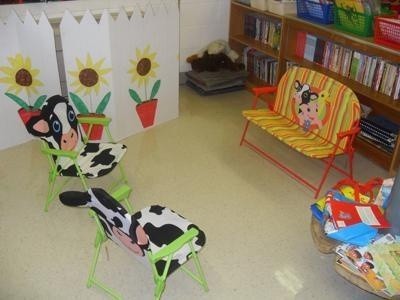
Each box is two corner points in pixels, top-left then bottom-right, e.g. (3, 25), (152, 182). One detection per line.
(290, 80), (323, 134)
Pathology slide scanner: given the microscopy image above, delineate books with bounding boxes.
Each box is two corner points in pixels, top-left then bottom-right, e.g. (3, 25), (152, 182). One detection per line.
(249, 0), (267, 11)
(267, 0), (297, 15)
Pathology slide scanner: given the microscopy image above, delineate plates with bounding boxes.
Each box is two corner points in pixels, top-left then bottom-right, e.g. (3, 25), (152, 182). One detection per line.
(229, 1), (283, 102)
(276, 15), (400, 178)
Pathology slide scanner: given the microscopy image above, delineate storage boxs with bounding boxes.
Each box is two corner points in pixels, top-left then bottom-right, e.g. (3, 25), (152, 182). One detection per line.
(239, 65), (362, 200)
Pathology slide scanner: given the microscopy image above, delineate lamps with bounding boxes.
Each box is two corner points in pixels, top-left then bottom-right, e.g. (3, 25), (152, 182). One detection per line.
(59, 183), (209, 300)
(27, 95), (127, 213)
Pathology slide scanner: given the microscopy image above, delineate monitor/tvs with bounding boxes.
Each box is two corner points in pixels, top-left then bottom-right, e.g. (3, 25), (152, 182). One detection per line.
(305, 0), (330, 21)
(295, 30), (400, 100)
(335, 0), (386, 31)
(310, 180), (391, 233)
(241, 47), (278, 87)
(358, 112), (399, 152)
(243, 12), (279, 49)
(335, 233), (400, 296)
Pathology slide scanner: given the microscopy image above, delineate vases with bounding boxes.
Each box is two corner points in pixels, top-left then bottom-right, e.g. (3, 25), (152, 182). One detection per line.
(0, 56), (48, 111)
(67, 54), (113, 114)
(129, 46), (160, 103)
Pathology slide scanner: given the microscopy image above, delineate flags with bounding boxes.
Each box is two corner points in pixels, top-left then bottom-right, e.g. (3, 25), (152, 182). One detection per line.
(17, 106), (40, 132)
(135, 99), (158, 126)
(77, 113), (105, 141)
